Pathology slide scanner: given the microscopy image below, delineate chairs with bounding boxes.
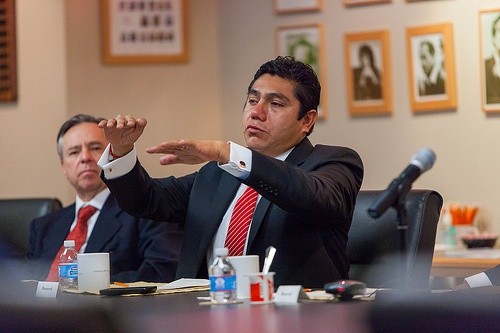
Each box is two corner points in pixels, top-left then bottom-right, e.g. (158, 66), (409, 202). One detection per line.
(0, 199), (63, 279)
(346, 189), (443, 289)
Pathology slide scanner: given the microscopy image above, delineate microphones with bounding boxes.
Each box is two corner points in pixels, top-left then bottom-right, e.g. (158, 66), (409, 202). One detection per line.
(367, 148), (436, 219)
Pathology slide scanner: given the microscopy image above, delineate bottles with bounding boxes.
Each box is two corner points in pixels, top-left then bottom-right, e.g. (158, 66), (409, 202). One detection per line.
(209, 247), (237, 302)
(57, 239), (79, 291)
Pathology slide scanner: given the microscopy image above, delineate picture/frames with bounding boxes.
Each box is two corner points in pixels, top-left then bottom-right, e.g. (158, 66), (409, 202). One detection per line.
(99, 0), (191, 66)
(269, 0), (500, 120)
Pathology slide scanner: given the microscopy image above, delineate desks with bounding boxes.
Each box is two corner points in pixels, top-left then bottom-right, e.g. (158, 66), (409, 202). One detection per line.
(0, 287), (500, 333)
(431, 242), (500, 288)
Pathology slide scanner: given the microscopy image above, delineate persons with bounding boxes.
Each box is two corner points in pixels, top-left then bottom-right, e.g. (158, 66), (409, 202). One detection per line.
(351, 39), (380, 100)
(416, 39), (446, 97)
(96, 55), (365, 289)
(24, 112), (171, 285)
(485, 16), (500, 105)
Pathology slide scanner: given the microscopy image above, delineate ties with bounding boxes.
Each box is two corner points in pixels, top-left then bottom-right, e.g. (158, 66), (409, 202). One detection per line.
(224, 186), (259, 255)
(45, 206), (97, 282)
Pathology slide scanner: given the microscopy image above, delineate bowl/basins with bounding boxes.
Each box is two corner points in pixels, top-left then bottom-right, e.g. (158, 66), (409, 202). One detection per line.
(462, 233), (497, 249)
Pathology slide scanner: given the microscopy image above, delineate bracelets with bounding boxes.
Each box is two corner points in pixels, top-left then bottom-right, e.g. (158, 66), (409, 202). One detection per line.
(109, 144), (133, 159)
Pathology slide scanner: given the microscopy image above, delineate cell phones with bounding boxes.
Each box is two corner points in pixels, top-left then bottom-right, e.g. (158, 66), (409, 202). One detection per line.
(98, 286), (158, 296)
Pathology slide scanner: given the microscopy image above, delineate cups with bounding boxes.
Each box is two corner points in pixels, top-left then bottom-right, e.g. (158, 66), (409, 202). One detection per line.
(454, 224), (480, 247)
(243, 272), (275, 301)
(77, 253), (110, 292)
(227, 256), (261, 298)
